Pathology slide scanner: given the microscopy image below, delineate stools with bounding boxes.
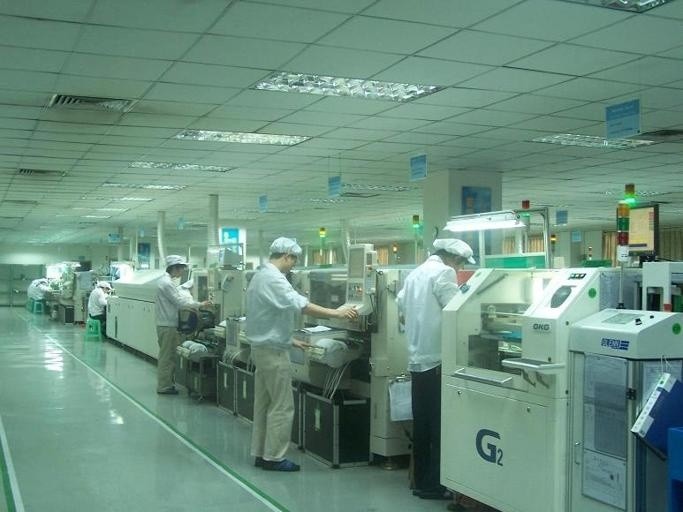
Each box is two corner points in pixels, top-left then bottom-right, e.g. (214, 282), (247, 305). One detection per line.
(85, 317), (102, 342)
(26, 295), (44, 315)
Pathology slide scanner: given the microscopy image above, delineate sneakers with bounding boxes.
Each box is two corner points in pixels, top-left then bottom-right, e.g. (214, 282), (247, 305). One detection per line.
(255, 457), (299, 471)
(157, 385), (177, 394)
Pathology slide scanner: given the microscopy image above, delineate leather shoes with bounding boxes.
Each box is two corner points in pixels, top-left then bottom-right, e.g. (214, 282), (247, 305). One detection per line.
(413, 488), (461, 498)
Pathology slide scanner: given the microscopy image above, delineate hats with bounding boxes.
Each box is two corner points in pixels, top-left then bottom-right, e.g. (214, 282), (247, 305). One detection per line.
(100, 281), (111, 288)
(166, 255), (189, 266)
(433, 239), (476, 264)
(270, 238), (302, 257)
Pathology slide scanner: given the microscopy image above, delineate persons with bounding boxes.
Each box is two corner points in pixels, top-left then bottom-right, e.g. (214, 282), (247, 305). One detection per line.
(396, 239), (477, 500)
(27, 278), (51, 315)
(155, 255), (213, 394)
(245, 237), (359, 471)
(89, 281), (112, 340)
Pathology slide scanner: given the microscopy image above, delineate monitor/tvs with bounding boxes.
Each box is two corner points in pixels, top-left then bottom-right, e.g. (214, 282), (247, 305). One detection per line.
(346, 244), (374, 283)
(628, 203), (660, 269)
(218, 247), (240, 270)
